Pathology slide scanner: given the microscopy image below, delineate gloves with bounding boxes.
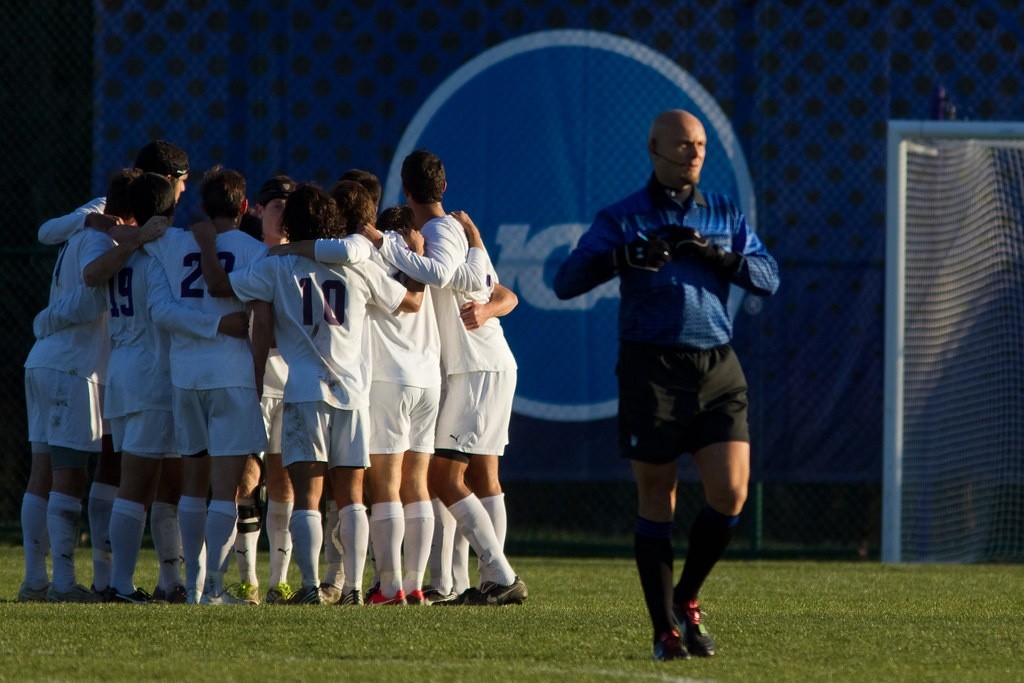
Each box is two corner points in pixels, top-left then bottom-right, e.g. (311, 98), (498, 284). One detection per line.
(670, 222), (727, 276)
(610, 233), (670, 274)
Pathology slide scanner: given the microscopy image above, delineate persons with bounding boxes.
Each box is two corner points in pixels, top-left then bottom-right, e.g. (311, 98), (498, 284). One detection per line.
(268, 150), (528, 606)
(17, 140), (295, 605)
(553, 108), (779, 662)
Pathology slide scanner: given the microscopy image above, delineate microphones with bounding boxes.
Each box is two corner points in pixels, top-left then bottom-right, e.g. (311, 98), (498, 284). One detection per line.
(655, 153), (685, 166)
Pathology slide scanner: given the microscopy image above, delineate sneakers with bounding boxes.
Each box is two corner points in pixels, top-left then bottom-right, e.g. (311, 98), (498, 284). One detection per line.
(321, 582), (342, 605)
(406, 589), (423, 605)
(363, 582), (407, 606)
(186, 590), (202, 604)
(46, 581), (91, 604)
(422, 585), (447, 606)
(266, 581), (292, 604)
(150, 583), (187, 605)
(446, 588), (460, 599)
(432, 587), (480, 606)
(17, 581), (51, 602)
(671, 589), (716, 657)
(653, 624), (691, 662)
(465, 576), (527, 607)
(230, 579), (260, 605)
(109, 586), (153, 605)
(198, 583), (247, 606)
(332, 589), (365, 607)
(284, 586), (324, 605)
(88, 583), (113, 603)
